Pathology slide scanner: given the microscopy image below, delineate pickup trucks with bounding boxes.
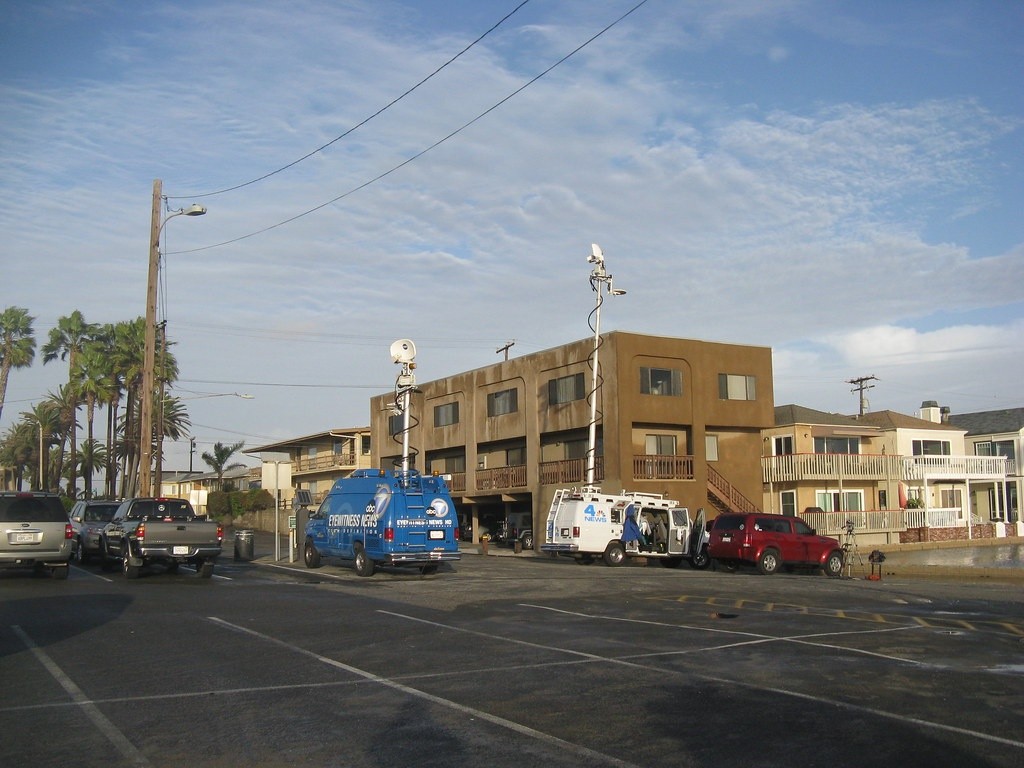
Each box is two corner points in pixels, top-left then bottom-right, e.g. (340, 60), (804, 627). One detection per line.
(98, 497), (222, 579)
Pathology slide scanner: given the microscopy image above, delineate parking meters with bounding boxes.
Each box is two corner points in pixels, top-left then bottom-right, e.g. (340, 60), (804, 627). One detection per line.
(288, 515), (296, 563)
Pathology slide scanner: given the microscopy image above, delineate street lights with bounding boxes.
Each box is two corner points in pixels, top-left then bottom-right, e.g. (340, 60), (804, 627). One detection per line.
(154, 394), (255, 500)
(263, 459), (294, 561)
(139, 203), (208, 494)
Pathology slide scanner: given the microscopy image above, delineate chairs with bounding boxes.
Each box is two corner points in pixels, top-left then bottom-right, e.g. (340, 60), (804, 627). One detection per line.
(653, 516), (668, 543)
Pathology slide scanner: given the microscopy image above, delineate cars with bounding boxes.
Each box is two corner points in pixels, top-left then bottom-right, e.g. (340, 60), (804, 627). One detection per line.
(502, 513), (533, 549)
(707, 513), (844, 577)
(462, 519), (497, 542)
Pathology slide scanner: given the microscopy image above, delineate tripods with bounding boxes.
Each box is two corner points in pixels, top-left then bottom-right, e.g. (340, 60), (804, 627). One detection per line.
(838, 523), (866, 579)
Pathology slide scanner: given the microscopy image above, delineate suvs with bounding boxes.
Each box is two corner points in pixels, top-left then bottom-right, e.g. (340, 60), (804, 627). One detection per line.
(0, 491), (73, 580)
(68, 499), (125, 561)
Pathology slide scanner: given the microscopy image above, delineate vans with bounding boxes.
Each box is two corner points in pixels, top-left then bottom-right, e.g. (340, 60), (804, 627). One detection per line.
(540, 487), (711, 571)
(303, 470), (462, 577)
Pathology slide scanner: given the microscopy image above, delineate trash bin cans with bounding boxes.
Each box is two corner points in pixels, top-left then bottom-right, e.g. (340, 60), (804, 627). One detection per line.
(234, 529), (254, 562)
